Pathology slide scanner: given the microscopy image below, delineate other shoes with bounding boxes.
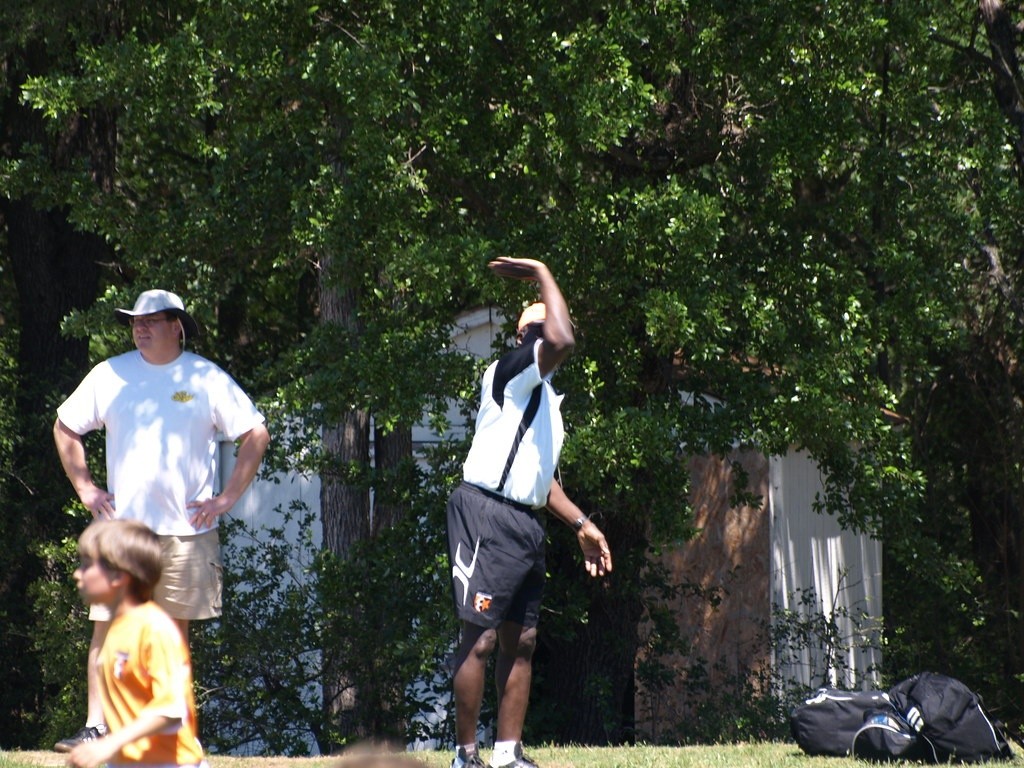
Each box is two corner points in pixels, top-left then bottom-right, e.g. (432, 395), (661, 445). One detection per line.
(451, 743), (486, 768)
(53, 726), (104, 751)
(487, 753), (539, 768)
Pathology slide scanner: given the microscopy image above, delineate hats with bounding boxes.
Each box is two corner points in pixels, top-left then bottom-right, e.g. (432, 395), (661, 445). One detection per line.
(113, 288), (201, 341)
(516, 302), (576, 333)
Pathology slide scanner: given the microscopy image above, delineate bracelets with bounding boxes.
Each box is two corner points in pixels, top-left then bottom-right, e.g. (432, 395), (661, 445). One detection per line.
(572, 514), (588, 533)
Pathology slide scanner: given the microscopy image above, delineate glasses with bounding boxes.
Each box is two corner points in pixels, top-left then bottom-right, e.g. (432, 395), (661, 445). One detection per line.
(128, 316), (168, 327)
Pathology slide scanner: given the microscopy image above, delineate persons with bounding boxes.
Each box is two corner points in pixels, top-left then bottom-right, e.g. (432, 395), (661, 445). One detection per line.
(53, 290), (270, 754)
(446, 257), (612, 768)
(65, 518), (210, 768)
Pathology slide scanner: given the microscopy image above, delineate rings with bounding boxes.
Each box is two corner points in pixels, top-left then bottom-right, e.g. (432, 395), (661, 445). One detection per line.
(201, 512), (206, 517)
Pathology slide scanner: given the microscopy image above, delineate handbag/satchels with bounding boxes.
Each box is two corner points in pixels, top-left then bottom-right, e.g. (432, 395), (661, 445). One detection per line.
(788, 670), (1016, 763)
